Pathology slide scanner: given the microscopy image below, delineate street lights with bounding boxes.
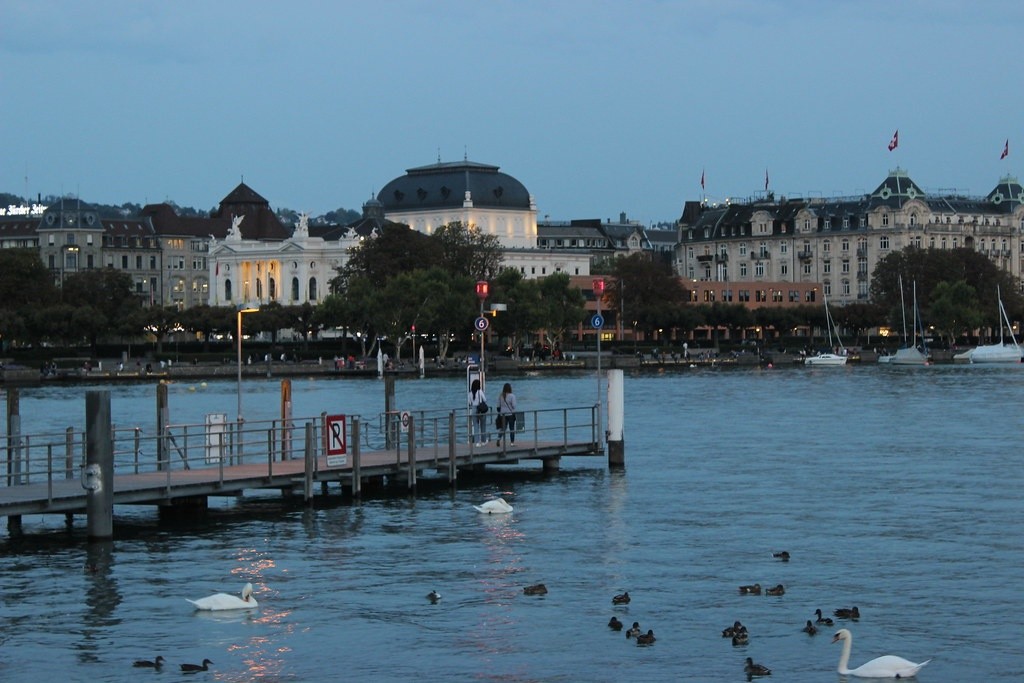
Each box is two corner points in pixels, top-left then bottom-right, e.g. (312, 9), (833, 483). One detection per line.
(236, 302), (260, 465)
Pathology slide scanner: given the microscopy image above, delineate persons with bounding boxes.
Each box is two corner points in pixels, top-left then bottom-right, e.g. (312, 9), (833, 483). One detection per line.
(43, 361), (49, 376)
(84, 361), (89, 376)
(952, 343), (958, 353)
(264, 352), (272, 367)
(636, 338), (890, 363)
(160, 360), (165, 370)
(468, 380), (488, 448)
(193, 358), (199, 366)
(539, 345), (548, 362)
(51, 361), (58, 376)
(280, 353), (287, 363)
(168, 359), (172, 368)
(136, 359), (141, 366)
(496, 383), (516, 447)
(334, 354), (356, 371)
(247, 355), (252, 366)
(117, 361), (123, 375)
(383, 352), (390, 368)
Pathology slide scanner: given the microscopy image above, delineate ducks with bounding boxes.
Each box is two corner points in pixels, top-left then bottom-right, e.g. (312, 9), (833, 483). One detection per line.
(721, 621), (749, 645)
(636, 629), (656, 645)
(626, 622), (641, 639)
(744, 657), (771, 675)
(766, 584), (784, 595)
(613, 592), (630, 605)
(133, 656), (165, 667)
(608, 617), (623, 631)
(180, 659), (213, 673)
(834, 605), (860, 618)
(803, 620), (816, 634)
(428, 589), (440, 597)
(815, 608), (833, 624)
(739, 583), (761, 592)
(524, 583), (548, 595)
(771, 551), (790, 559)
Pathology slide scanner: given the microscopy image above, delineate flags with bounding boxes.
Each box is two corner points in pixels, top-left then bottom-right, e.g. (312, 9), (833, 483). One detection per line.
(765, 170), (769, 190)
(701, 170), (705, 189)
(1000, 139), (1008, 160)
(888, 130), (898, 151)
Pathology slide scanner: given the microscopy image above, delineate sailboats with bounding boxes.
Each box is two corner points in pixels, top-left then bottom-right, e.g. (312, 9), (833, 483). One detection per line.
(954, 283), (1024, 365)
(877, 273), (935, 365)
(804, 293), (849, 365)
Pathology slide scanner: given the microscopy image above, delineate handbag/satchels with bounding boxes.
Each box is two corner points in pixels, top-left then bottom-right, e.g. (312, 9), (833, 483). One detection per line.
(495, 415), (503, 430)
(513, 415), (516, 420)
(478, 402), (488, 413)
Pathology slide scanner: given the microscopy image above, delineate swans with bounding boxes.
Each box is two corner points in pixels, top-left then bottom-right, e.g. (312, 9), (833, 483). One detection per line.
(832, 629), (933, 678)
(473, 498), (514, 514)
(184, 582), (258, 610)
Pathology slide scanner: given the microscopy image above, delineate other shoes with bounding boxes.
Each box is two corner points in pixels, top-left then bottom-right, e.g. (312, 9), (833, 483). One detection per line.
(496, 440), (500, 447)
(476, 442), (482, 447)
(510, 443), (514, 447)
(482, 439), (488, 445)
